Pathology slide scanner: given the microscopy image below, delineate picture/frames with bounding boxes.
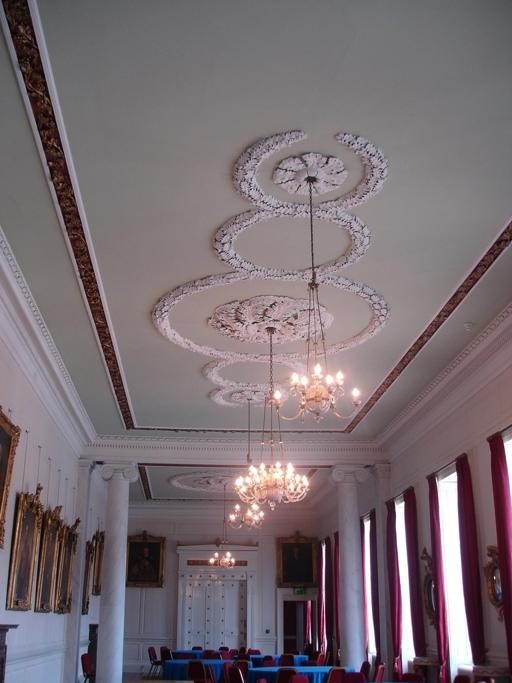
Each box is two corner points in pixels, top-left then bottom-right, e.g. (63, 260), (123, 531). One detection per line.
(277, 530), (317, 588)
(420, 547), (438, 630)
(0, 405), (104, 615)
(484, 546), (505, 621)
(125, 531), (167, 588)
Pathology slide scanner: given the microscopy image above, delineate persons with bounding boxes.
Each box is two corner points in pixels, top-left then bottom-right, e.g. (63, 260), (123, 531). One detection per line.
(130, 545), (156, 582)
(287, 544), (308, 580)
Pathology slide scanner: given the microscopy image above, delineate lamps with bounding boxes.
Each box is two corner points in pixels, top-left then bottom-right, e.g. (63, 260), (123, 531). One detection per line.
(227, 390), (264, 529)
(209, 480), (235, 569)
(270, 171), (362, 423)
(232, 322), (309, 505)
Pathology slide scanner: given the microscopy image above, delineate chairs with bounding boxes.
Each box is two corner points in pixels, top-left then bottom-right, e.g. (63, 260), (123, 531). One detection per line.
(146, 645), (495, 682)
(81, 653), (96, 683)
